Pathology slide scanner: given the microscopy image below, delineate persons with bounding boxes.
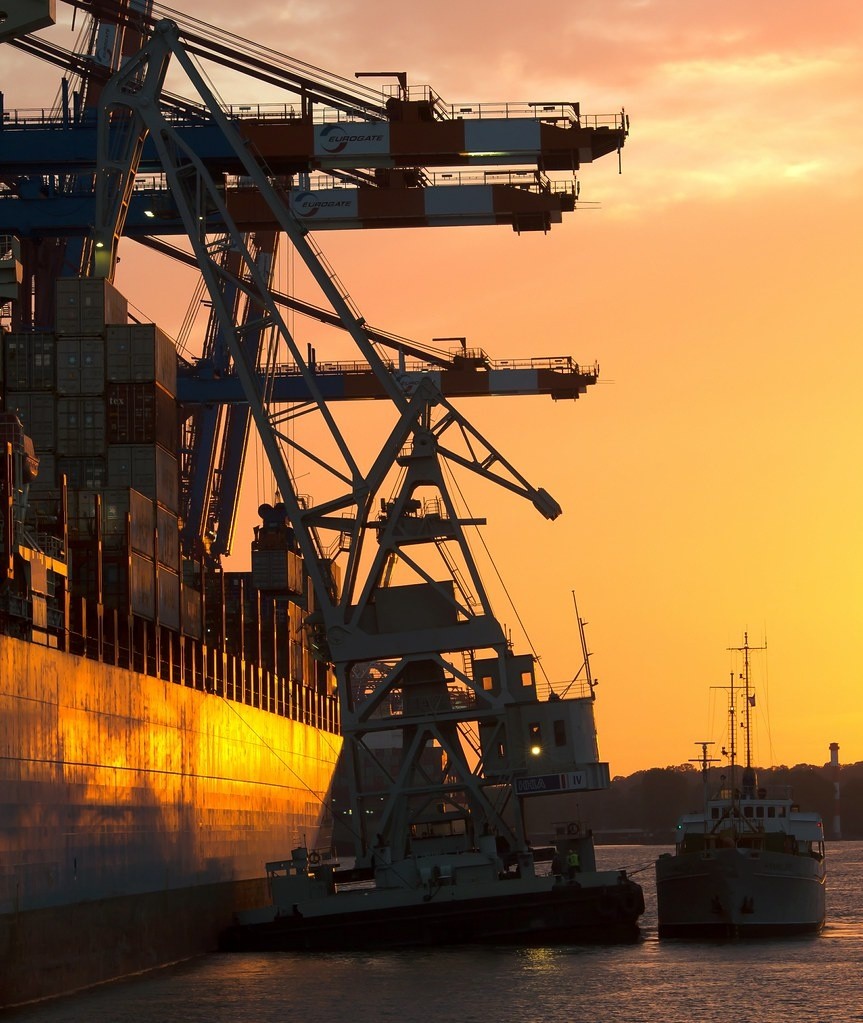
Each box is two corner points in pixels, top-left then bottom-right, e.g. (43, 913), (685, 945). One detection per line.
(567, 848), (581, 877)
(721, 832), (735, 848)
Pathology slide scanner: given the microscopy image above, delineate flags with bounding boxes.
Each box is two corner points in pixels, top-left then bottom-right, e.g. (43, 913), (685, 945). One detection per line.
(748, 693), (756, 707)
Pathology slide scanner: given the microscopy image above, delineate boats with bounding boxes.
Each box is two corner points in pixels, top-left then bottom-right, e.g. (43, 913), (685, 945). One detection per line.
(654, 626), (828, 939)
